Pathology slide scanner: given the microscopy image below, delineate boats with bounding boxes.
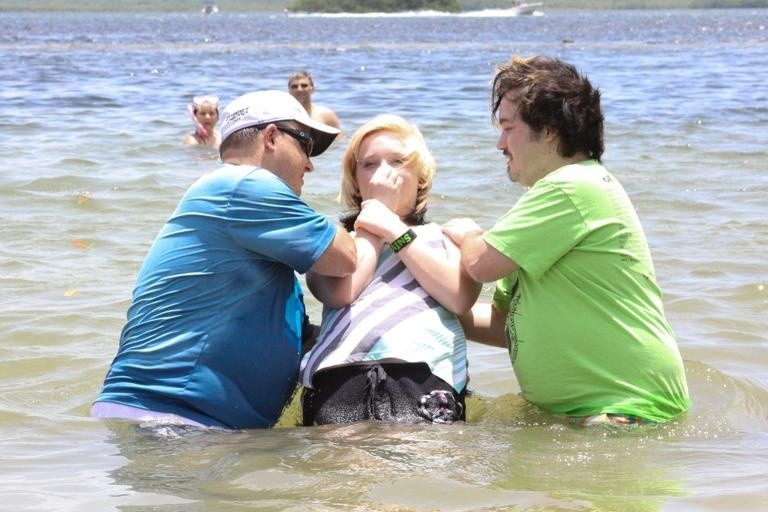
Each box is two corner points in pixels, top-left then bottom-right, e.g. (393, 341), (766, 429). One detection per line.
(513, 3), (543, 16)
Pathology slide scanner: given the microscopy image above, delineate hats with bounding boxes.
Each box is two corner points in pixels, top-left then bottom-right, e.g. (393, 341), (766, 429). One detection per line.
(213, 90), (341, 157)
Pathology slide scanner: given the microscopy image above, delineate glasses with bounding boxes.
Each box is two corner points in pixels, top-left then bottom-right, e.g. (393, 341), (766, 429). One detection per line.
(256, 124), (314, 158)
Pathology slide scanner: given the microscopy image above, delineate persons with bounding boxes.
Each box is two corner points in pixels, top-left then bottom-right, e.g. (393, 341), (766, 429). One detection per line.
(298, 111), (482, 425)
(441, 50), (691, 427)
(88, 89), (359, 427)
(288, 70), (345, 139)
(181, 94), (222, 149)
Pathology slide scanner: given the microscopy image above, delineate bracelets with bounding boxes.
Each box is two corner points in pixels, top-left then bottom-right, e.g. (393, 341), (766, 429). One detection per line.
(390, 229), (417, 252)
